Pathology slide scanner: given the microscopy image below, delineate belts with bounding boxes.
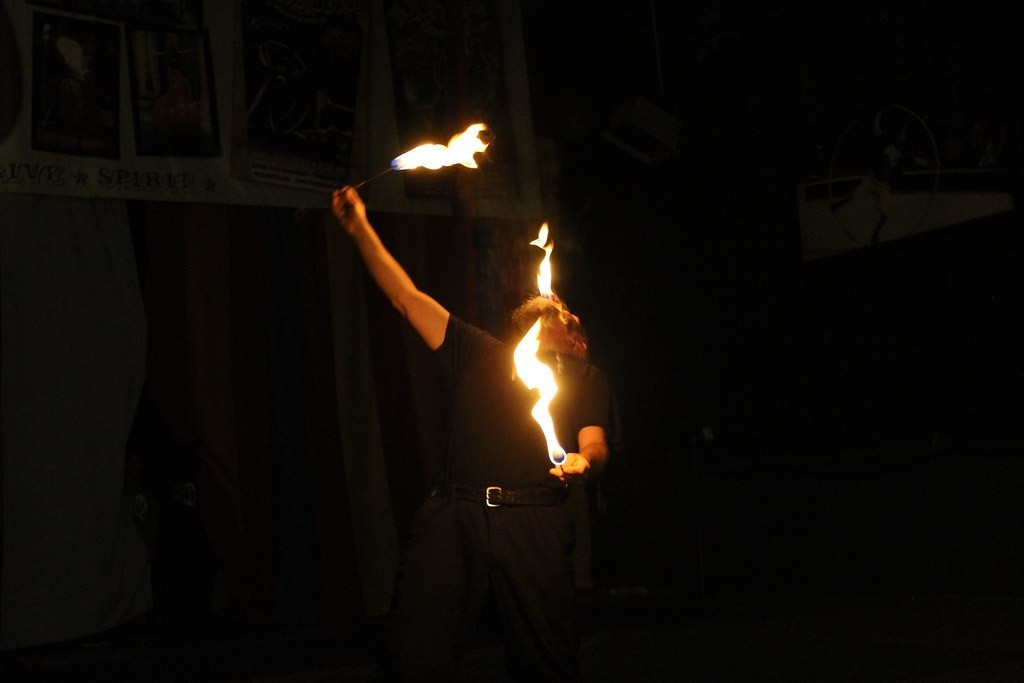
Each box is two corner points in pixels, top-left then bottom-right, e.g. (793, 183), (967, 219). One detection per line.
(436, 483), (552, 509)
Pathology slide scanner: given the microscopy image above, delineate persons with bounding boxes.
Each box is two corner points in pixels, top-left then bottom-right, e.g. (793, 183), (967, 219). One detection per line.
(332, 186), (610, 683)
